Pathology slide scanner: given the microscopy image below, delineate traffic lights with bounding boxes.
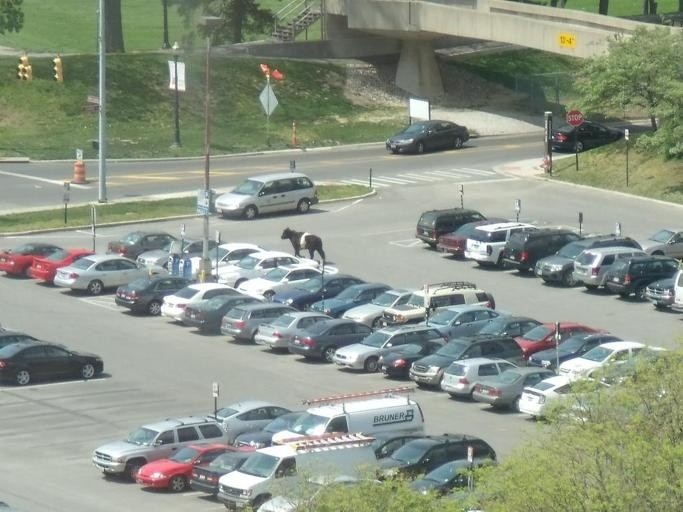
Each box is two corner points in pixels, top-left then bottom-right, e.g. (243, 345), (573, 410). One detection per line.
(50, 57), (61, 85)
(15, 63), (21, 81)
(17, 55), (28, 65)
(22, 66), (31, 81)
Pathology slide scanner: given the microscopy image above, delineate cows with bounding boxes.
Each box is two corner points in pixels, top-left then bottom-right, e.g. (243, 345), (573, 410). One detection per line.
(280, 227), (324, 259)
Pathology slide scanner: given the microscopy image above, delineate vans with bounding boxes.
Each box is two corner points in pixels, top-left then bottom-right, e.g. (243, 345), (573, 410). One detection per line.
(211, 173), (322, 220)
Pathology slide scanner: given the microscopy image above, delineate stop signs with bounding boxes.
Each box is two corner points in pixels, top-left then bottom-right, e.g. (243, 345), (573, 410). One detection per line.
(563, 108), (585, 128)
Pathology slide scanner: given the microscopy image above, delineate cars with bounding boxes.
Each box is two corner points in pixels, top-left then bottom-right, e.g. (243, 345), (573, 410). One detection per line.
(385, 117), (468, 156)
(547, 120), (624, 153)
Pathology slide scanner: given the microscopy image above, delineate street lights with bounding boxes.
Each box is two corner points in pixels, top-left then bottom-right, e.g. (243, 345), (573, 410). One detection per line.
(167, 41), (185, 150)
